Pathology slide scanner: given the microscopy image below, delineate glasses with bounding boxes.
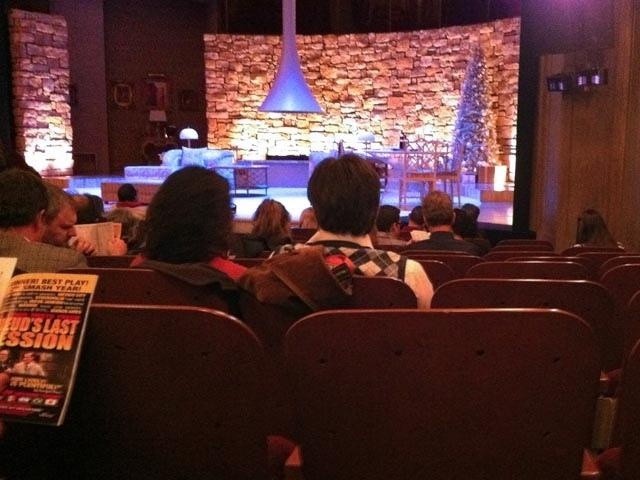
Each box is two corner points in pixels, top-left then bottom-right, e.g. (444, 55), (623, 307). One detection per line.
(227, 203), (237, 213)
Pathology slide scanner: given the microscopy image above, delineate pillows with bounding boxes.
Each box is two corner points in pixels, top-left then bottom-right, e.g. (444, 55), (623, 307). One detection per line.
(180, 145), (207, 169)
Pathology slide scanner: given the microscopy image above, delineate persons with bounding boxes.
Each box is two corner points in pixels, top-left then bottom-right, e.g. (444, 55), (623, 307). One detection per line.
(127, 164), (249, 281)
(401, 205), (427, 234)
(375, 205), (405, 246)
(405, 190), (488, 254)
(570, 208), (626, 251)
(291, 208), (319, 241)
(265, 152), (435, 309)
(1, 168), (89, 276)
(233, 198), (293, 260)
(39, 183), (151, 256)
(6, 351), (44, 378)
(0, 350), (10, 373)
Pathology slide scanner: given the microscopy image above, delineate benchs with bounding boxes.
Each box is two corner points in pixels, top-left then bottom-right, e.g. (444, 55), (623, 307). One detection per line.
(101, 179), (165, 204)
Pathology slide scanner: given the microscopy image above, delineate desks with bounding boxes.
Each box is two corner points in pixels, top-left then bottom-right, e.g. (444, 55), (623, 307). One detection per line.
(232, 165), (270, 196)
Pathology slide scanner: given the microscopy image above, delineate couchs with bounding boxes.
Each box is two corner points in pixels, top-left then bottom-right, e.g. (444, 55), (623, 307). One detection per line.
(124, 147), (234, 182)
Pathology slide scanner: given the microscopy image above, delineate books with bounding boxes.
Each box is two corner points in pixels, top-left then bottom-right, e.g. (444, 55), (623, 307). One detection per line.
(1, 273), (101, 426)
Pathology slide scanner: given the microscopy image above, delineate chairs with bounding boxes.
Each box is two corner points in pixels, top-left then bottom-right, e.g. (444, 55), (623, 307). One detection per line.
(57, 238), (640, 480)
(338, 137), (465, 209)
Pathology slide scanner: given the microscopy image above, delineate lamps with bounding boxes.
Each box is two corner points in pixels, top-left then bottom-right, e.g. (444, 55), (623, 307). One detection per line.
(179, 126), (198, 148)
